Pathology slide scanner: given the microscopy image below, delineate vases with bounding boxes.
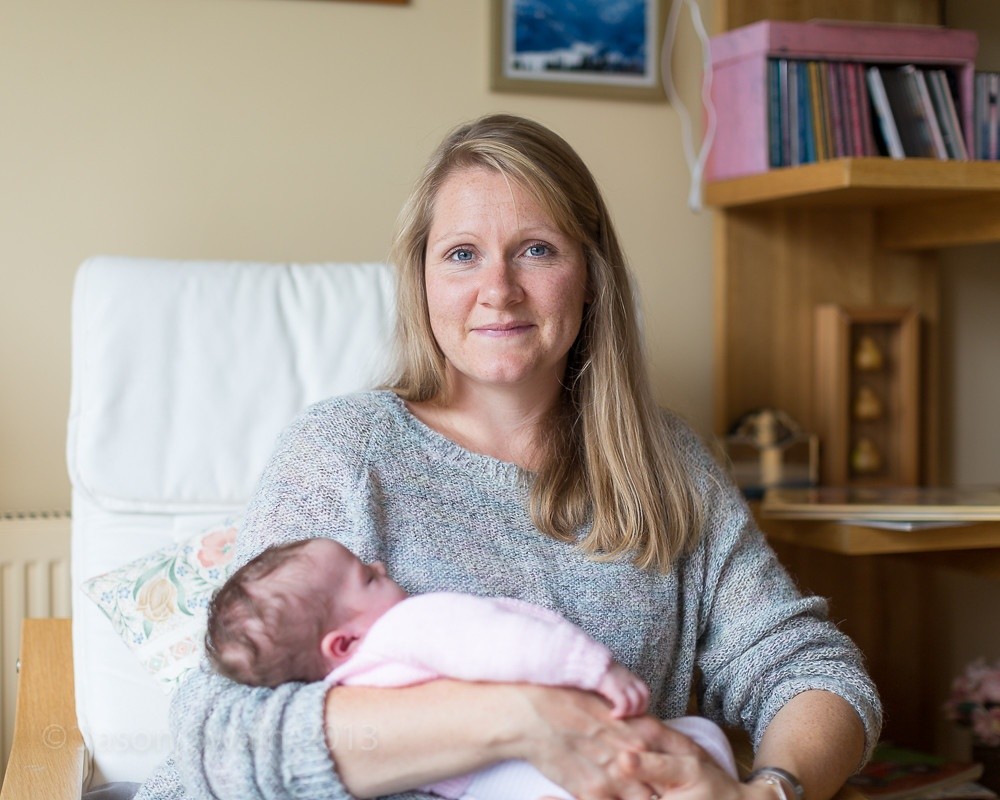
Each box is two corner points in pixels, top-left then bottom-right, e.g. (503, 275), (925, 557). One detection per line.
(972, 738), (1000, 799)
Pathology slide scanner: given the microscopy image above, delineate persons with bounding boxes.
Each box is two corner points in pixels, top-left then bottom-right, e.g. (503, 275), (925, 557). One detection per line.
(203, 532), (738, 799)
(119, 115), (887, 800)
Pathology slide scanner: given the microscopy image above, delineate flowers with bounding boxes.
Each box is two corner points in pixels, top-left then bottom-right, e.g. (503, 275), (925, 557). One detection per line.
(939, 660), (1000, 746)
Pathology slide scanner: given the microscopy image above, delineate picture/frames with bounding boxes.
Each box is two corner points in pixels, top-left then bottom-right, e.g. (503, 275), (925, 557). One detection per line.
(483, 0), (683, 104)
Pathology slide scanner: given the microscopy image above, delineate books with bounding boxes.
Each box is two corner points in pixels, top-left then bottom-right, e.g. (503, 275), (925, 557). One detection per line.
(707, 16), (1000, 184)
(757, 464), (1000, 534)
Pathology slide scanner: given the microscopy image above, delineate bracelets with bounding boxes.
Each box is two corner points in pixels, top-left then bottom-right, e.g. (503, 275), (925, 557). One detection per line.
(751, 764), (805, 800)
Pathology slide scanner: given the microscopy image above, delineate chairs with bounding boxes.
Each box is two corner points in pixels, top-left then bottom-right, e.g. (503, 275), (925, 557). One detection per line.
(60, 248), (468, 799)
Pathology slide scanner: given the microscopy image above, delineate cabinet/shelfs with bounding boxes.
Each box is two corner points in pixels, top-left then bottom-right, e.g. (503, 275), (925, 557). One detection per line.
(705, 1), (999, 799)
